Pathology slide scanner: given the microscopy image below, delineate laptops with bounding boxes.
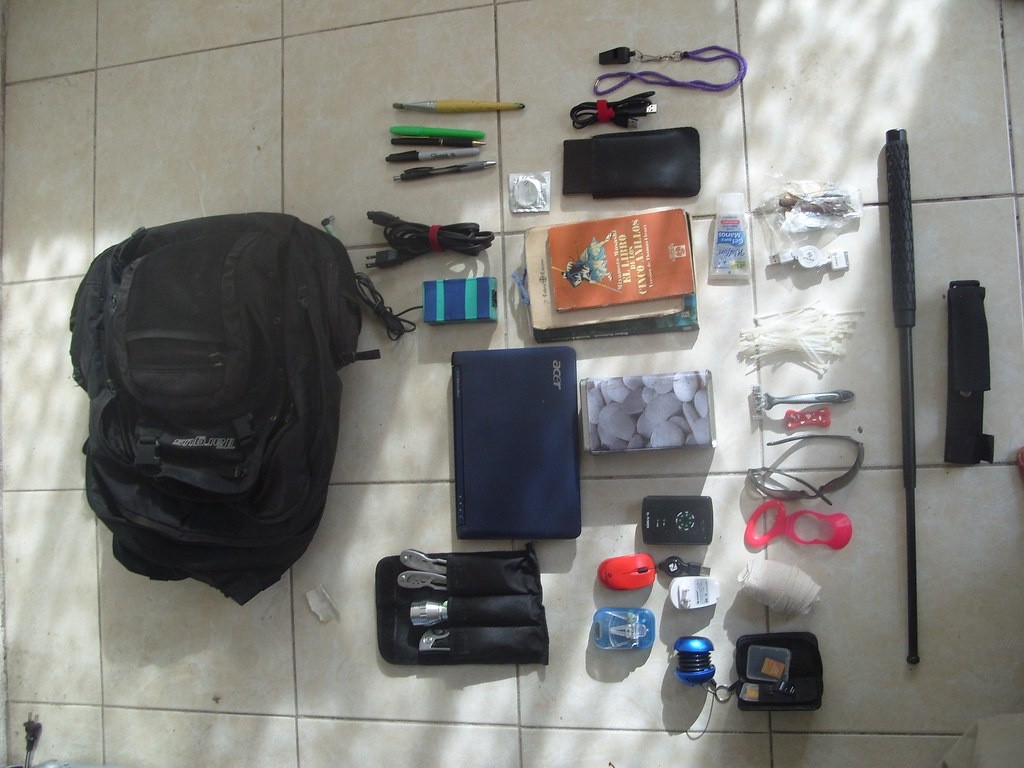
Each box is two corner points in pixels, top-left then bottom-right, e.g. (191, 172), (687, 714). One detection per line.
(451, 345), (582, 539)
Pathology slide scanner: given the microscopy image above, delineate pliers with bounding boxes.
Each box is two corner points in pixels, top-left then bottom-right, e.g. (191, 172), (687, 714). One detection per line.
(397, 548), (447, 574)
(397, 570), (448, 591)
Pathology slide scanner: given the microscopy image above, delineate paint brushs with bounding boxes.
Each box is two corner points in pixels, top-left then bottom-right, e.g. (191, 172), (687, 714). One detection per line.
(391, 101), (526, 110)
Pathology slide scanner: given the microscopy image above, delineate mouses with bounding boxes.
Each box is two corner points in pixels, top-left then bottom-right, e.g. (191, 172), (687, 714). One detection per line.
(598, 552), (656, 590)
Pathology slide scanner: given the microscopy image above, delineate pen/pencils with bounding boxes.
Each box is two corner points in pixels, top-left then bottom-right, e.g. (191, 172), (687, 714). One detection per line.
(389, 126), (486, 139)
(385, 148), (482, 163)
(393, 161), (496, 181)
(390, 137), (487, 149)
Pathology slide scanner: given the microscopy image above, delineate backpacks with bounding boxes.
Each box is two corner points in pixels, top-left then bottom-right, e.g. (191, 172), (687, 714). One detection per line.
(71, 213), (362, 605)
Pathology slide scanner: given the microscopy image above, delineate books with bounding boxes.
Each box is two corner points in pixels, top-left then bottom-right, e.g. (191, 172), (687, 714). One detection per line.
(511, 207), (700, 345)
